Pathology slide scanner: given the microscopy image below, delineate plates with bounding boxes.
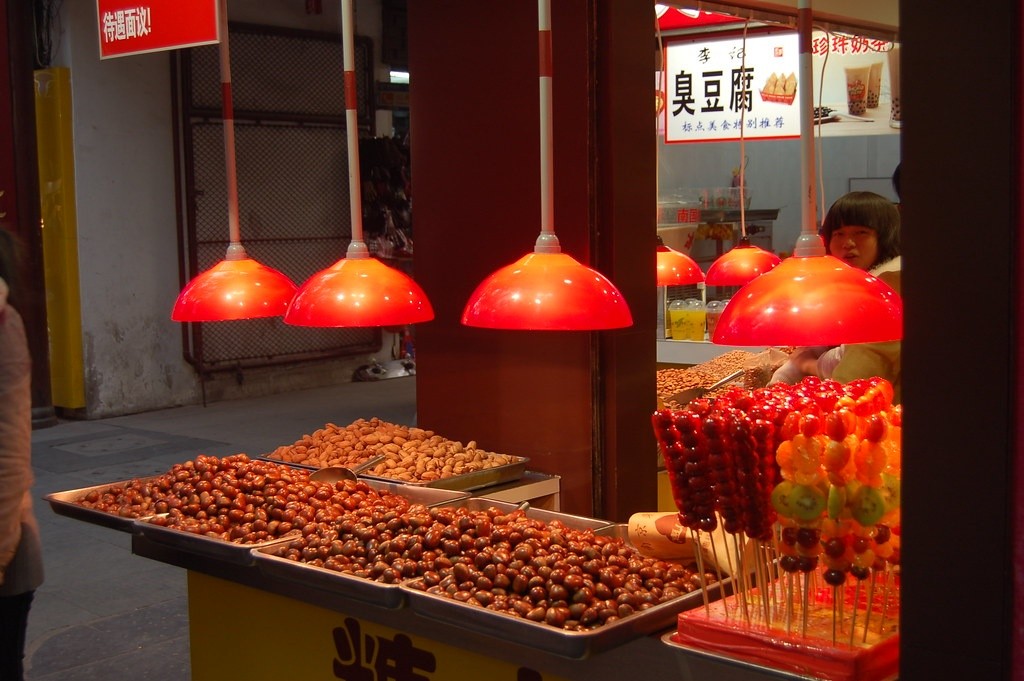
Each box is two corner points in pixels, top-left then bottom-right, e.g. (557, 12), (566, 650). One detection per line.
(814, 117), (835, 123)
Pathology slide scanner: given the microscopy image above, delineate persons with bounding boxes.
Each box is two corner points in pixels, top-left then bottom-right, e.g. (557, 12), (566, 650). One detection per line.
(765, 163), (905, 410)
(0, 274), (46, 680)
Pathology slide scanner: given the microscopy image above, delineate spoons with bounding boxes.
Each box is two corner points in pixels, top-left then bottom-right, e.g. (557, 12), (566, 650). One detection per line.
(828, 111), (875, 122)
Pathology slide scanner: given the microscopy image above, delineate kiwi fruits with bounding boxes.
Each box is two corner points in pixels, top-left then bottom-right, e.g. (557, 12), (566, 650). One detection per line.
(773, 475), (900, 525)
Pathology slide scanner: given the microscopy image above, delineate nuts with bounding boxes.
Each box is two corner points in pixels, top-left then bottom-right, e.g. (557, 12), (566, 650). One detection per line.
(267, 415), (520, 483)
(658, 348), (759, 407)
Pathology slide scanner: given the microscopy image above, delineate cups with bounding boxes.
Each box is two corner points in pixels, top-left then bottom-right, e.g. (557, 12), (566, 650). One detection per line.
(665, 299), (706, 341)
(844, 65), (871, 115)
(706, 302), (723, 342)
(888, 42), (901, 129)
(867, 59), (883, 108)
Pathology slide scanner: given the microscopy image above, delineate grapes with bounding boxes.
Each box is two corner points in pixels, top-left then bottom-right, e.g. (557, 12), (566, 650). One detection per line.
(777, 527), (899, 585)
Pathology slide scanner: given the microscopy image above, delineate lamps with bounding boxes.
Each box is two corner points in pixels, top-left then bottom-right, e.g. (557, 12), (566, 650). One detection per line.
(168, 0), (904, 350)
